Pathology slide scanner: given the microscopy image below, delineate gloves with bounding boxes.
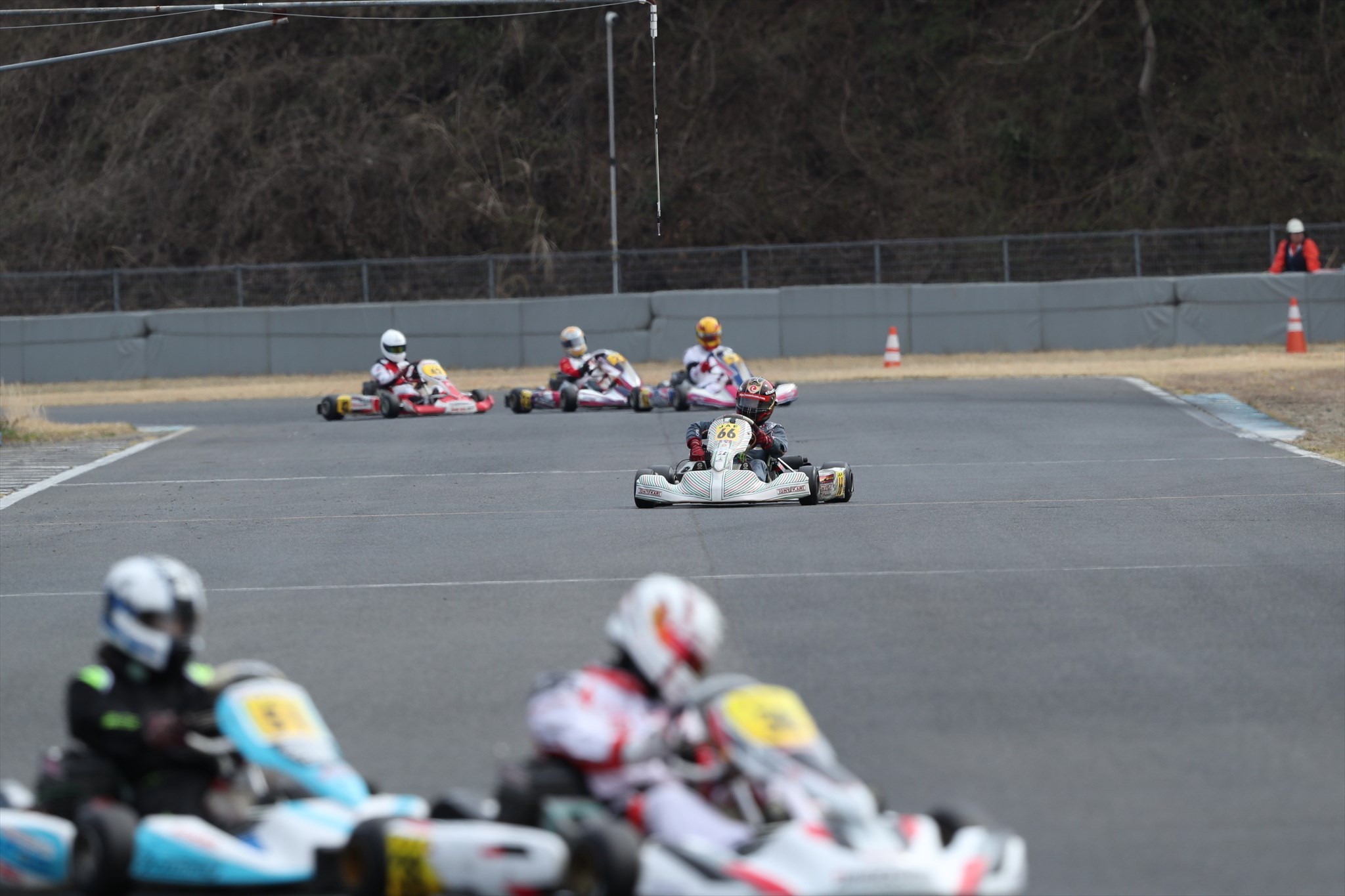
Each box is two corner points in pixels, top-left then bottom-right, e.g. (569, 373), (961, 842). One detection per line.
(753, 428), (773, 450)
(622, 703), (713, 767)
(687, 437), (706, 462)
(143, 707), (206, 755)
(396, 364), (414, 379)
(700, 356), (717, 373)
(579, 359), (598, 377)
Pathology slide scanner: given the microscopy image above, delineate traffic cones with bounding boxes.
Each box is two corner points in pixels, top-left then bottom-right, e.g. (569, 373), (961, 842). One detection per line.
(882, 326), (901, 368)
(1285, 296), (1307, 354)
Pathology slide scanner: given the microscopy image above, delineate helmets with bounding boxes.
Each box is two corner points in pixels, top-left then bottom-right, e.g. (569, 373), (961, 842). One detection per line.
(735, 377), (776, 426)
(603, 571), (726, 704)
(380, 329), (407, 363)
(696, 316), (722, 352)
(97, 555), (207, 671)
(1286, 218), (1305, 233)
(560, 326), (588, 357)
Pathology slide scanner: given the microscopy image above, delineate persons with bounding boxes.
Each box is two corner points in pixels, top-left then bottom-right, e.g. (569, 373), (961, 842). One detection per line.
(683, 316), (733, 387)
(1267, 218), (1321, 274)
(559, 326), (617, 393)
(687, 377), (788, 482)
(369, 328), (438, 404)
(496, 573), (725, 861)
(65, 552), (246, 831)
